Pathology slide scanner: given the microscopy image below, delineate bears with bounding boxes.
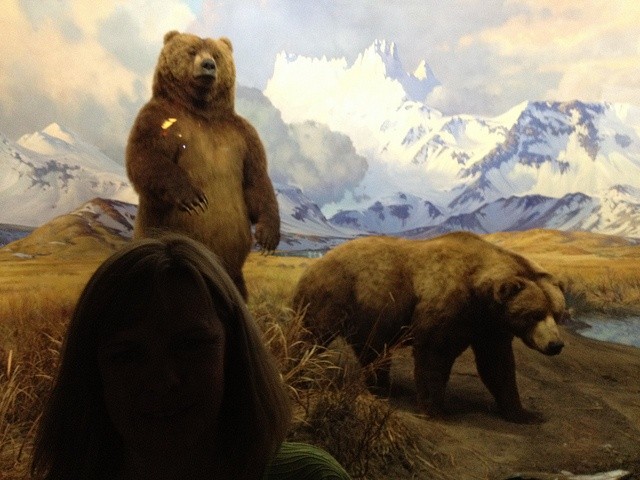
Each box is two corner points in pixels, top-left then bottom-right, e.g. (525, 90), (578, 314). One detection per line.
(282, 231), (565, 422)
(125, 30), (280, 305)
